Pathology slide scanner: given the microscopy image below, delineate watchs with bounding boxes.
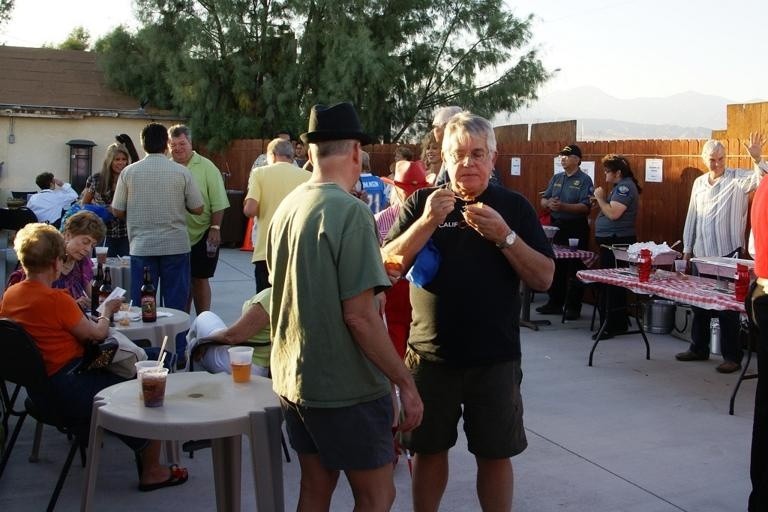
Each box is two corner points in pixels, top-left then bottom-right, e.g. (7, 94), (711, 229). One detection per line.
(495, 231), (516, 249)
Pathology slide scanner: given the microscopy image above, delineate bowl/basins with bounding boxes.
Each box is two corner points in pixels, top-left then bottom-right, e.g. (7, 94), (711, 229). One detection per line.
(463, 202), (483, 221)
(385, 254), (404, 274)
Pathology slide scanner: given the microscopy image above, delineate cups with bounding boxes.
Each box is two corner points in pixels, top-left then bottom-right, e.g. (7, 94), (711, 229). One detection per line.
(95, 246), (108, 265)
(207, 239), (217, 258)
(228, 346), (254, 382)
(135, 360), (168, 408)
(674, 260), (687, 277)
(569, 238), (579, 251)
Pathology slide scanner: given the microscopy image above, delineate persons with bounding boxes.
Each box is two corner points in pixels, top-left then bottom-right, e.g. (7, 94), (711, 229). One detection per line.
(2, 122), (231, 492)
(591, 154), (642, 340)
(264, 101), (425, 512)
(536, 145), (595, 320)
(379, 110), (556, 512)
(184, 105), (465, 479)
(747, 171), (768, 512)
(675, 131), (768, 373)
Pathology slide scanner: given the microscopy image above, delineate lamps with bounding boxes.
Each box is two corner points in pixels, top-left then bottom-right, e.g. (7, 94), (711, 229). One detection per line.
(9, 116), (16, 144)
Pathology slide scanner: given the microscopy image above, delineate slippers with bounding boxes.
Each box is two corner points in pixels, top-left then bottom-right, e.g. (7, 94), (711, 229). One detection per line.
(137, 462), (189, 492)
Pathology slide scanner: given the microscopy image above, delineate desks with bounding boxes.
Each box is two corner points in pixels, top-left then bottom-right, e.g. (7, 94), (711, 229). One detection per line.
(575, 268), (759, 415)
(520, 243), (600, 331)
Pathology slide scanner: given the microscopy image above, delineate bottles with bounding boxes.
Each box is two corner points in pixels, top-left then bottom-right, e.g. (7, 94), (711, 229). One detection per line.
(90, 264), (113, 324)
(638, 248), (652, 283)
(734, 262), (751, 302)
(141, 267), (156, 323)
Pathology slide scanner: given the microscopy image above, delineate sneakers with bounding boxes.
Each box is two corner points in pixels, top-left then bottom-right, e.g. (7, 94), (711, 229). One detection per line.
(675, 346), (710, 361)
(565, 299), (582, 320)
(717, 359), (742, 373)
(535, 299), (563, 315)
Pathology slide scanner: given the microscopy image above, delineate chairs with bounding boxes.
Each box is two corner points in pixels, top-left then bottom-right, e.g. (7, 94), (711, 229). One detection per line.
(181, 337), (291, 463)
(0, 206), (62, 271)
(1, 317), (120, 512)
(561, 244), (618, 331)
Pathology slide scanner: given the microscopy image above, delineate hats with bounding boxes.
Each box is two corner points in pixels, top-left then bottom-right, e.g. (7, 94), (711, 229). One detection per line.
(394, 161), (428, 194)
(556, 145), (582, 157)
(299, 101), (372, 147)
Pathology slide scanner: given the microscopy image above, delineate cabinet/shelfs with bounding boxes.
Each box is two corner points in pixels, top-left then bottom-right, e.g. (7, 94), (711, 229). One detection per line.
(612, 244), (683, 271)
(690, 252), (755, 283)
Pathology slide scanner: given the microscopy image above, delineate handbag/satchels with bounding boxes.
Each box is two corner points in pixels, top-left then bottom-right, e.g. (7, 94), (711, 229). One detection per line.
(59, 201), (115, 234)
(93, 325), (148, 380)
